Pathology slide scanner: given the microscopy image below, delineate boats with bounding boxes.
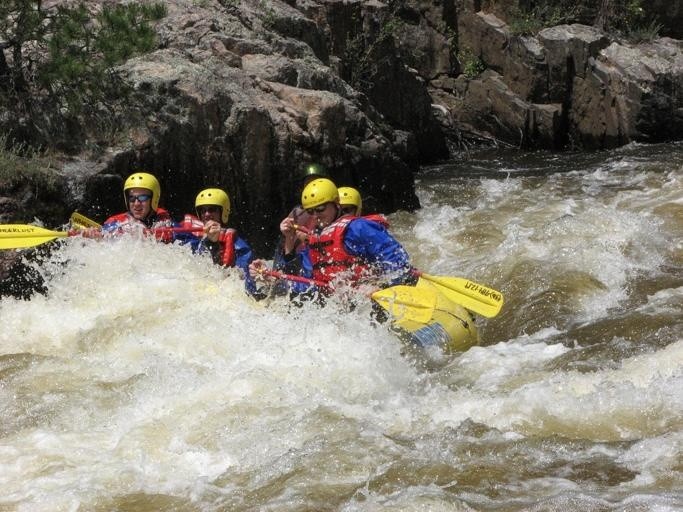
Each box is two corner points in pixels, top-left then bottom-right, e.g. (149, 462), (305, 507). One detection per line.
(386, 275), (478, 372)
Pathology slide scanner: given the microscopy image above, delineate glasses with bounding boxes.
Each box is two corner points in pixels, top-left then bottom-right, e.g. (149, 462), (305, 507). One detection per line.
(199, 207), (217, 214)
(129, 195), (152, 202)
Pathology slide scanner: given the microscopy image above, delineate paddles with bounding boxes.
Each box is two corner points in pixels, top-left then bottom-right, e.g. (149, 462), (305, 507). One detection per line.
(250, 264), (437, 323)
(0, 224), (206, 249)
(409, 270), (504, 318)
(71, 212), (101, 230)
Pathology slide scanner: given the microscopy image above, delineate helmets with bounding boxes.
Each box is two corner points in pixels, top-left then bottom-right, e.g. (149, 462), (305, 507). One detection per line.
(301, 163), (362, 217)
(124, 172), (161, 212)
(195, 188), (230, 224)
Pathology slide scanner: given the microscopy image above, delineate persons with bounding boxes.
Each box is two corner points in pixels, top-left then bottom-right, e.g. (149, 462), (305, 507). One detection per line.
(247, 183), (388, 306)
(270, 162), (330, 275)
(172, 187), (257, 296)
(103, 171), (221, 264)
(278, 177), (416, 325)
